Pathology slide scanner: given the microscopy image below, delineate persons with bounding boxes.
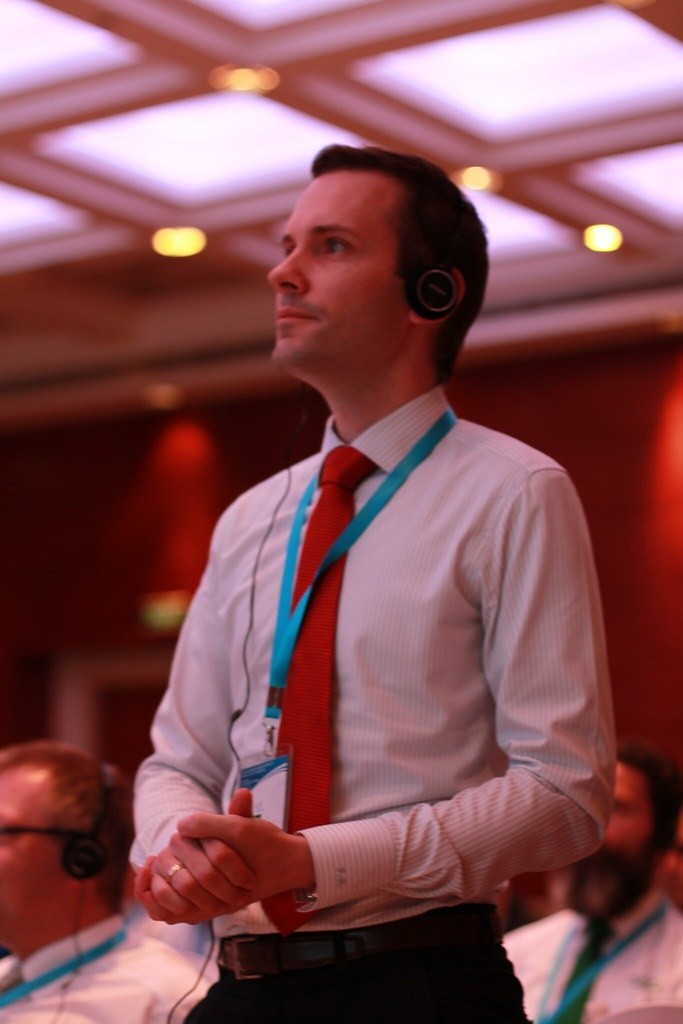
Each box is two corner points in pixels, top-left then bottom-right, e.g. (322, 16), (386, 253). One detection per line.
(0, 745), (683, 1023)
(0, 746), (220, 1024)
(129, 147), (616, 1024)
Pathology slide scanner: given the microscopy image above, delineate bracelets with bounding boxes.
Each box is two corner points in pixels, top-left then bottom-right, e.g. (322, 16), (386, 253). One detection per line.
(295, 888), (317, 902)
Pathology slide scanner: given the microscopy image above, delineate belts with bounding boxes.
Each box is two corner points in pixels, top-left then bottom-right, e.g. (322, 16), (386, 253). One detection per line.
(218, 908), (495, 979)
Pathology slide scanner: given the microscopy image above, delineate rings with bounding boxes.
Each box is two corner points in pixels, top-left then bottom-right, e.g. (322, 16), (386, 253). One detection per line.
(166, 864), (187, 883)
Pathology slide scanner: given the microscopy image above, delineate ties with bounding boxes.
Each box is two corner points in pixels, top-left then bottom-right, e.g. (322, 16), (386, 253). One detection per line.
(260, 445), (377, 936)
(557, 918), (612, 1024)
(1, 966), (20, 991)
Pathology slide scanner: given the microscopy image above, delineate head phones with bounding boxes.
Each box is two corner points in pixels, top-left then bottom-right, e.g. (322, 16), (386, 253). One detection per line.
(59, 773), (116, 882)
(406, 201), (474, 320)
